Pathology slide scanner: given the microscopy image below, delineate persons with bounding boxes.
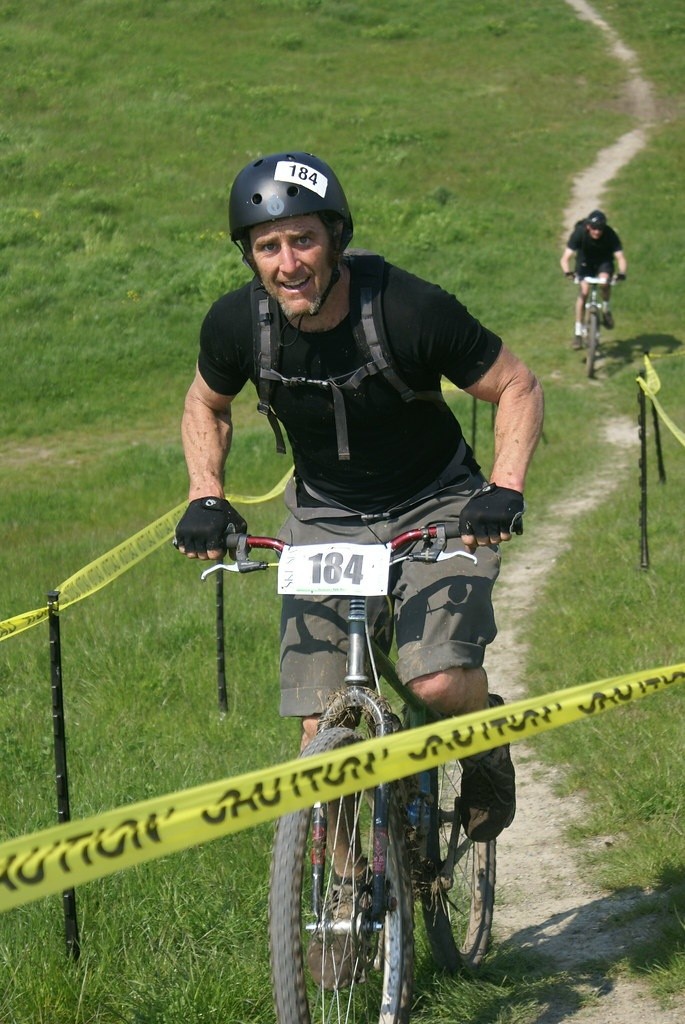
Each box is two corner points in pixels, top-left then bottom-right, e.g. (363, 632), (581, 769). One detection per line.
(175, 152), (543, 991)
(560, 210), (627, 351)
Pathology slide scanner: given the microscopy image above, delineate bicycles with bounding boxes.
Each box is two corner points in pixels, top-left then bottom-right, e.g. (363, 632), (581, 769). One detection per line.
(573, 271), (620, 378)
(175, 514), (524, 1024)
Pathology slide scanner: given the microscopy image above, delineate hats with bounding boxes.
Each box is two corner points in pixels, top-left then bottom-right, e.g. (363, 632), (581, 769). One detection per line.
(587, 211), (606, 228)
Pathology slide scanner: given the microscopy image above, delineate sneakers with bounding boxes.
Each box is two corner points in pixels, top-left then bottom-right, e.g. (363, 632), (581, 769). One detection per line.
(307, 858), (373, 990)
(460, 694), (516, 843)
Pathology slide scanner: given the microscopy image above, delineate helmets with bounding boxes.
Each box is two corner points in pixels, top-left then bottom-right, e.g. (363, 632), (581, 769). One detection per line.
(229, 152), (353, 252)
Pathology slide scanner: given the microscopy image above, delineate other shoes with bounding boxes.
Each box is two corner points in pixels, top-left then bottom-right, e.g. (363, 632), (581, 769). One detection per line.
(603, 311), (614, 329)
(573, 336), (582, 349)
